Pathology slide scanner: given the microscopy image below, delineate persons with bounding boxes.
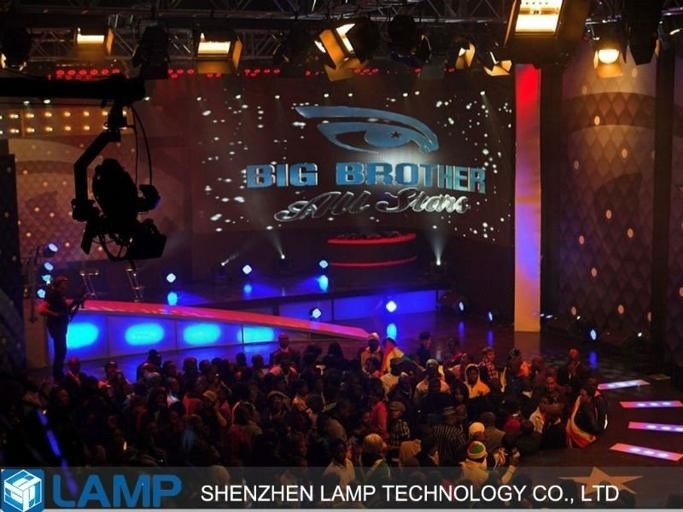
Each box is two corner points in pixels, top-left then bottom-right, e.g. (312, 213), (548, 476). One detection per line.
(37, 274), (87, 378)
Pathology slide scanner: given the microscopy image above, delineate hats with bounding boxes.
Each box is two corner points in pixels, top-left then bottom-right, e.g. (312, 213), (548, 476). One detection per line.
(502, 419), (521, 433)
(362, 434), (387, 453)
(465, 441), (488, 459)
(441, 406), (457, 416)
(200, 389), (217, 402)
(467, 421), (485, 434)
(386, 400), (406, 413)
(367, 332), (380, 343)
(420, 331), (431, 338)
